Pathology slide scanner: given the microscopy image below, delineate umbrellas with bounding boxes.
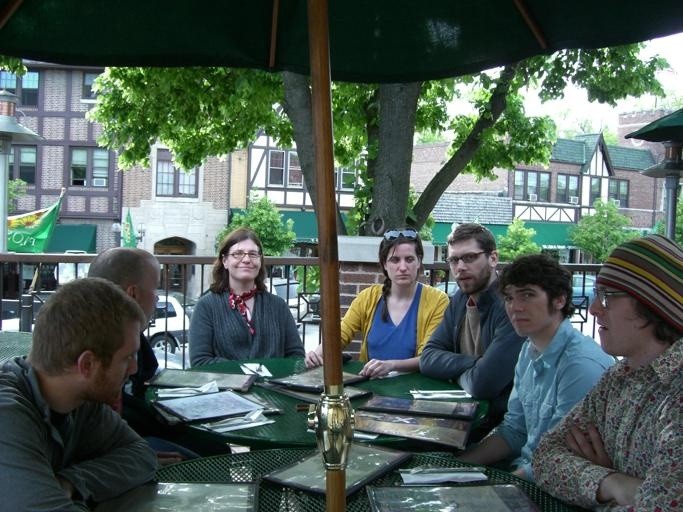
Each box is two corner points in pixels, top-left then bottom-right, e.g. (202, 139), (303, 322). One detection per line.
(0, 0), (683, 512)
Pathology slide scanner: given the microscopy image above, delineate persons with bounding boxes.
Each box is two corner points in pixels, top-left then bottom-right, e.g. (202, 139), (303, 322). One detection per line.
(419, 222), (527, 440)
(0, 276), (156, 511)
(459, 253), (616, 483)
(80, 247), (202, 466)
(189, 225), (305, 367)
(305, 226), (450, 381)
(531, 232), (683, 512)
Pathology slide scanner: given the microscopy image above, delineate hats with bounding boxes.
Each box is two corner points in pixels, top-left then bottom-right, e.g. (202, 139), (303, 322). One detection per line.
(596, 233), (683, 332)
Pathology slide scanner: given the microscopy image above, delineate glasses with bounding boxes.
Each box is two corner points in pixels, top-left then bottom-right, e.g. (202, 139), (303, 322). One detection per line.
(593, 289), (630, 309)
(446, 250), (489, 268)
(383, 227), (418, 242)
(226, 250), (261, 260)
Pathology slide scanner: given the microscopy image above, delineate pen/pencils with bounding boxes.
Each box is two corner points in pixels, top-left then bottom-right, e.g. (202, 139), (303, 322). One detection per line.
(240, 362), (261, 377)
(256, 361), (263, 373)
(393, 466), (488, 473)
(296, 405), (310, 412)
(404, 390), (467, 396)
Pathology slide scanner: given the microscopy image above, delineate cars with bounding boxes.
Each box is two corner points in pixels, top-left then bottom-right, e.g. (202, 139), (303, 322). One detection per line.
(569, 274), (596, 308)
(142, 295), (190, 354)
(264, 276), (312, 329)
(432, 282), (459, 301)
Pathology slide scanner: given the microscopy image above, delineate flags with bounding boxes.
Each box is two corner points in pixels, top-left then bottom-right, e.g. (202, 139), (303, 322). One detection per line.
(120, 206), (141, 248)
(7, 199), (61, 253)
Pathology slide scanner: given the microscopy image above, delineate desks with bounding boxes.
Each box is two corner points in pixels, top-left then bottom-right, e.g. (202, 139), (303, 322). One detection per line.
(79, 354), (594, 511)
(0, 331), (34, 369)
